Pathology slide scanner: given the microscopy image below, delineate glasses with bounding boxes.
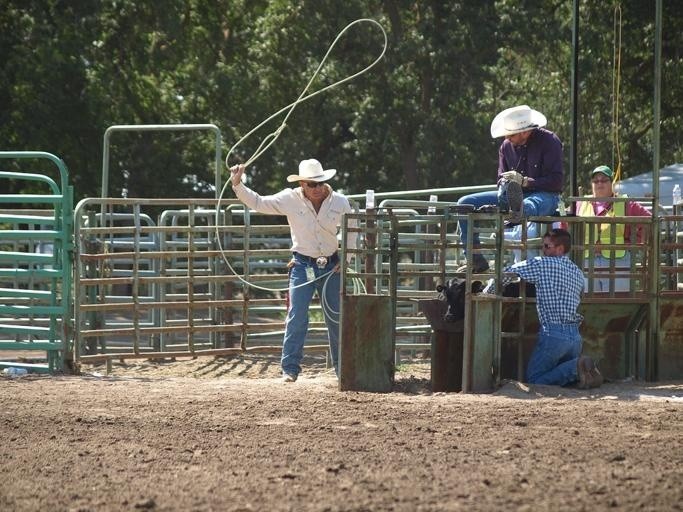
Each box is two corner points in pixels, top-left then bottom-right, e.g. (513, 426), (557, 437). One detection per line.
(306, 182), (324, 187)
(544, 243), (555, 248)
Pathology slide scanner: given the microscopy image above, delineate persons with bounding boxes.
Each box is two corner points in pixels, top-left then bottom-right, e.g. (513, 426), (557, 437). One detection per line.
(227, 156), (352, 385)
(490, 217), (560, 262)
(482, 226), (604, 393)
(453, 103), (567, 280)
(568, 165), (654, 294)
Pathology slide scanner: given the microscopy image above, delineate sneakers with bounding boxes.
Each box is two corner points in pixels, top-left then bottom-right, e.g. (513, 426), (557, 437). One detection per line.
(577, 356), (593, 389)
(282, 368), (297, 382)
(588, 357), (602, 388)
(456, 258), (488, 273)
(505, 181), (523, 224)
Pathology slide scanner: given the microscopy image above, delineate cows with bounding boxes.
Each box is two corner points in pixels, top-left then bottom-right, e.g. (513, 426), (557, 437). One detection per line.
(436, 277), (537, 324)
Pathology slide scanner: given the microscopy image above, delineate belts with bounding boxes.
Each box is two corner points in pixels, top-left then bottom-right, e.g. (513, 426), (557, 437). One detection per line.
(294, 252), (338, 270)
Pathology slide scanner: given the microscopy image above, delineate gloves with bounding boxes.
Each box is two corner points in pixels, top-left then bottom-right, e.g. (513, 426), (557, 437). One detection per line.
(501, 170), (528, 188)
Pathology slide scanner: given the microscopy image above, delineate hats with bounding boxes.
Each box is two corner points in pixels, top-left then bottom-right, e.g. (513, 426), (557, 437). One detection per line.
(286, 159), (336, 183)
(490, 105), (548, 139)
(591, 165), (612, 179)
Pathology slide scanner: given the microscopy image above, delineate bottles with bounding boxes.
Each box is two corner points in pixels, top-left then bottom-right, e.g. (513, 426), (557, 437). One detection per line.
(672, 184), (682, 205)
(3, 367), (27, 375)
